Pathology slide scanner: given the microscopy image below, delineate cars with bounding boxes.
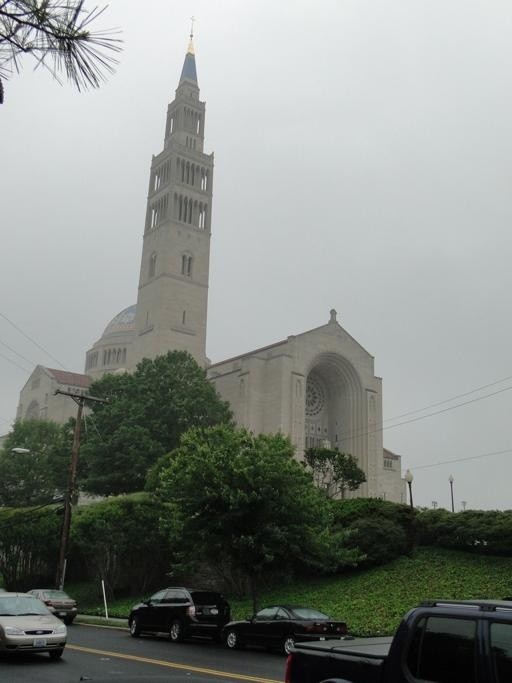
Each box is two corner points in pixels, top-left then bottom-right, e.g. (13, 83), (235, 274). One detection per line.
(27, 587), (78, 625)
(0, 591), (68, 662)
(222, 602), (353, 656)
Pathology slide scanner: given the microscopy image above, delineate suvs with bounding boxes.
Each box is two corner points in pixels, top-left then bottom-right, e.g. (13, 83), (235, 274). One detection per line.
(129, 585), (233, 641)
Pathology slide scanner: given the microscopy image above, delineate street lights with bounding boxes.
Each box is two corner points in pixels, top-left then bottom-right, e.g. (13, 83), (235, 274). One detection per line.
(404, 468), (414, 509)
(10, 442), (82, 588)
(448, 473), (455, 513)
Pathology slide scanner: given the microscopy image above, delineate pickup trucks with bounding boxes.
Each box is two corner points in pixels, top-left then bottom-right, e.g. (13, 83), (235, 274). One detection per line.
(278, 594), (511, 683)
